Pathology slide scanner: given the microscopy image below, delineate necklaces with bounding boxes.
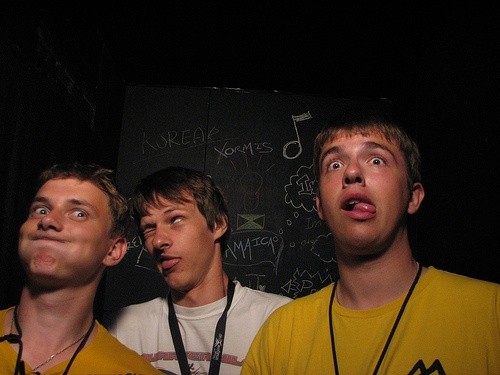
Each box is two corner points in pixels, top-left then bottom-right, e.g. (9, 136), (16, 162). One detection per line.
(0, 302), (95, 375)
(329, 259), (422, 375)
(168, 279), (235, 375)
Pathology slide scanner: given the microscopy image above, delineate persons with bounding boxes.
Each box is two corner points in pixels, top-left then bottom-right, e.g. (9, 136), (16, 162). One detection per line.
(0, 159), (165, 375)
(239, 107), (500, 375)
(102, 166), (294, 375)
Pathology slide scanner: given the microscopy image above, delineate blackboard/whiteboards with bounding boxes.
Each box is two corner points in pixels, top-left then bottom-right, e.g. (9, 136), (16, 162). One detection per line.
(105, 82), (443, 314)
